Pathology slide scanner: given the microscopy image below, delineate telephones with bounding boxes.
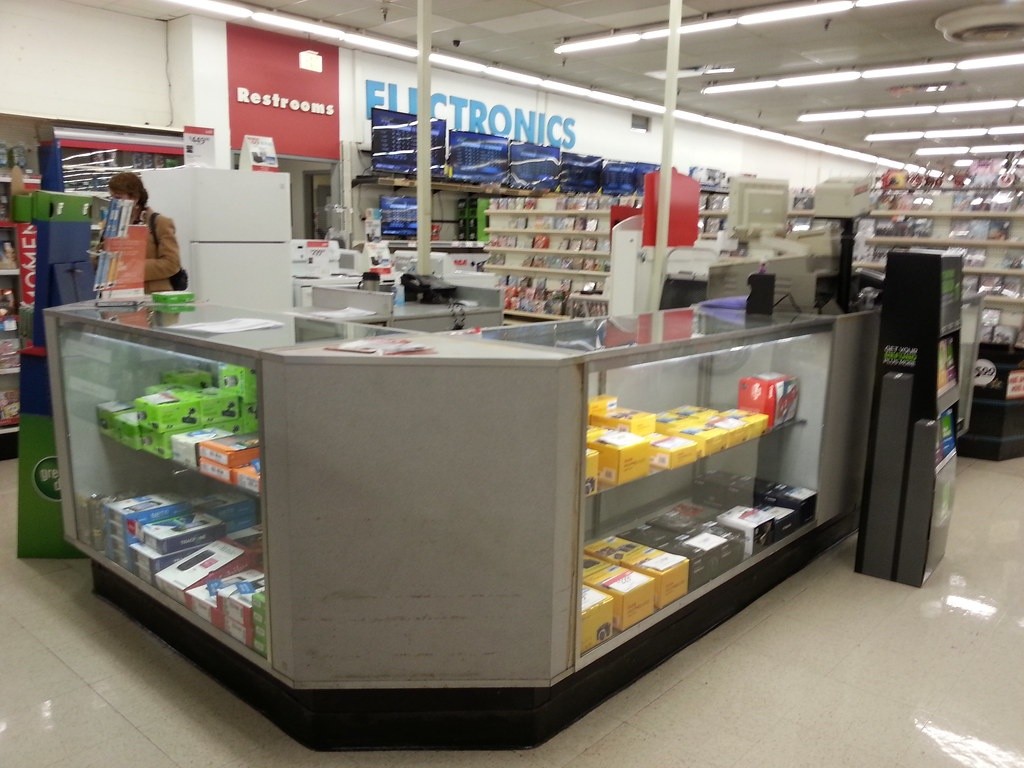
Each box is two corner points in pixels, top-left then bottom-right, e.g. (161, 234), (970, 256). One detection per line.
(400, 273), (458, 297)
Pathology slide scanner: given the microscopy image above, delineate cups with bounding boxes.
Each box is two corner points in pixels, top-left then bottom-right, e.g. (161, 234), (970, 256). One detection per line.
(357, 272), (380, 293)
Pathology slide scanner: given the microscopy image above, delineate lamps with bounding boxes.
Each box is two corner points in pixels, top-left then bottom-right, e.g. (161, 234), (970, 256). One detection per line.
(294, 30), (323, 74)
(553, 0), (1024, 165)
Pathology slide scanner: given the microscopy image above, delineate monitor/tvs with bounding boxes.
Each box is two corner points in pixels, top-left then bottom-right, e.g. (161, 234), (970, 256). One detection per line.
(727, 176), (790, 242)
(371, 109), (661, 197)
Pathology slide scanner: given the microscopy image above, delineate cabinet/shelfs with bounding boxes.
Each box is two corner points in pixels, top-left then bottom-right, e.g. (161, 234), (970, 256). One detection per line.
(853, 177), (1024, 348)
(483, 205), (612, 328)
(0, 174), (44, 463)
(43, 291), (880, 752)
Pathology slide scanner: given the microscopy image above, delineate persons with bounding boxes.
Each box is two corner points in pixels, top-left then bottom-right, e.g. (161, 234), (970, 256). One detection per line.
(109, 172), (181, 293)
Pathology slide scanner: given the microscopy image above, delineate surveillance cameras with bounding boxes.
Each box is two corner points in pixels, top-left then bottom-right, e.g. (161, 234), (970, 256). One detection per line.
(453, 40), (460, 47)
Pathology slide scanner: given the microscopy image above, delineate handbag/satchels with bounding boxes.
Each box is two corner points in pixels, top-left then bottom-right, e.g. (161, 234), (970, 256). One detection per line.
(168, 268), (188, 291)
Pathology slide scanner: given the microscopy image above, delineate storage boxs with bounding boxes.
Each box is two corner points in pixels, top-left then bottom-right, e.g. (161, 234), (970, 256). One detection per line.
(574, 371), (818, 653)
(74, 365), (268, 666)
